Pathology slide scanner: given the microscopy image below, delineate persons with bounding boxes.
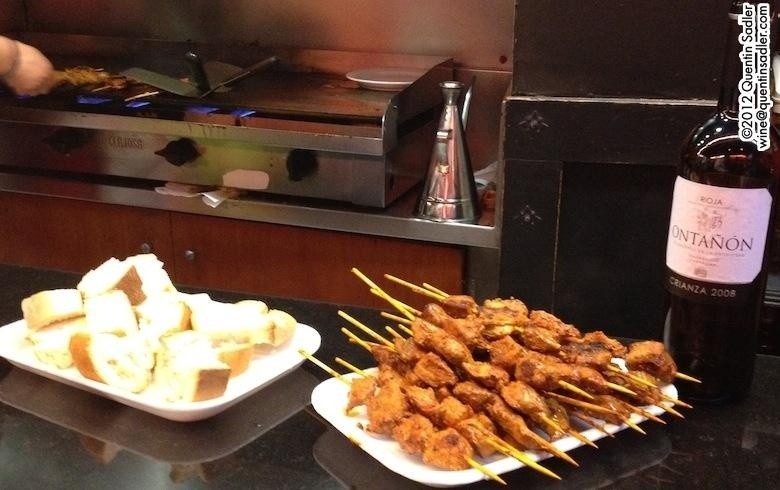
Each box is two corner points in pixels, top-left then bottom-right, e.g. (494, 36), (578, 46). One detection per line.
(1, 35), (60, 99)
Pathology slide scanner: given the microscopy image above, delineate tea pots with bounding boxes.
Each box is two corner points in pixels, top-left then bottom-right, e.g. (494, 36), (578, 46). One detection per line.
(412, 74), (483, 225)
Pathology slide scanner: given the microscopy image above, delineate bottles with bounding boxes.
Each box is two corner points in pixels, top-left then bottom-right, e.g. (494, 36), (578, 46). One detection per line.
(652, 1), (779, 408)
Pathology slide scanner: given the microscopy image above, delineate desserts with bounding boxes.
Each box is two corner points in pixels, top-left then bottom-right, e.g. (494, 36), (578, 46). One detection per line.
(21, 251), (297, 400)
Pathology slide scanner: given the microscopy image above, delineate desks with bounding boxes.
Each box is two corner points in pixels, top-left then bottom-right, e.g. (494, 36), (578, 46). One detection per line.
(2, 263), (778, 490)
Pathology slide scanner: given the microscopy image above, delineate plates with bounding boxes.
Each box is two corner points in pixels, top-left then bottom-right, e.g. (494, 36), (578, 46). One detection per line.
(346, 64), (425, 91)
(0, 293), (320, 421)
(312, 429), (674, 489)
(310, 356), (682, 489)
(0, 363), (323, 463)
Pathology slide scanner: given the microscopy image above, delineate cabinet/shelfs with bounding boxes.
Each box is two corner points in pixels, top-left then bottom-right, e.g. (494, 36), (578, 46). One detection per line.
(0, 189), (467, 321)
